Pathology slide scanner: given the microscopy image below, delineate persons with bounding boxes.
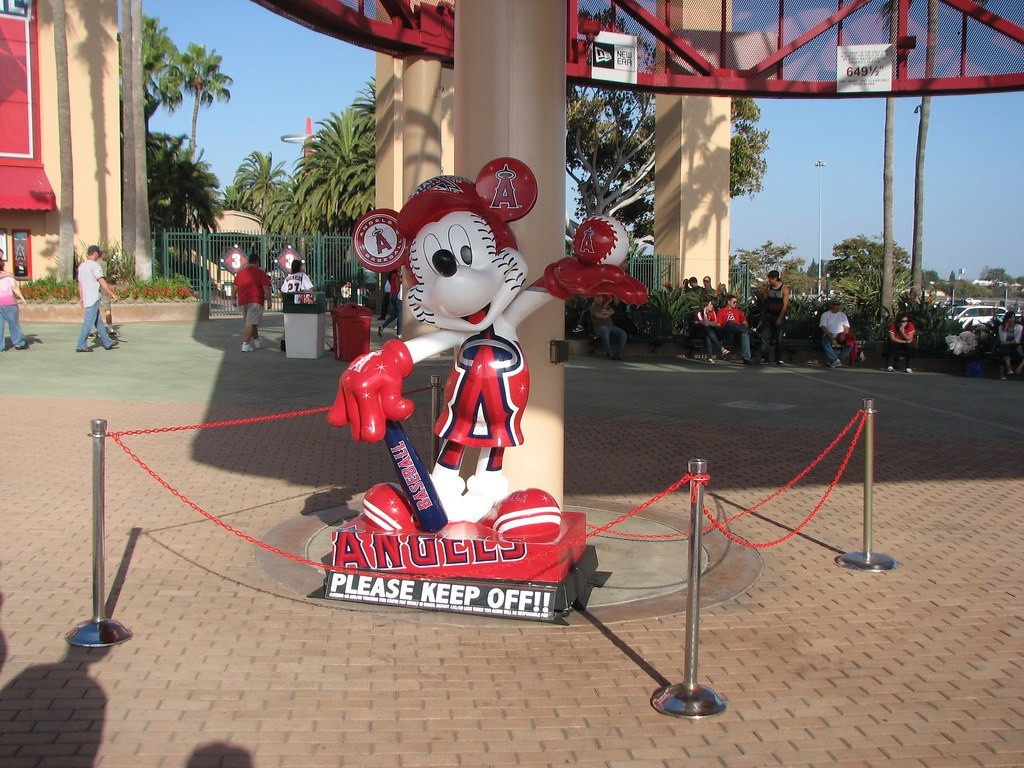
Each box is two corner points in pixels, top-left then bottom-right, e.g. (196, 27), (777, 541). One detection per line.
(377, 268), (402, 339)
(571, 290), (629, 362)
(998, 311), (1024, 379)
(75, 245), (121, 352)
(818, 300), (854, 369)
(717, 295), (757, 365)
(280, 260), (316, 350)
(885, 312), (916, 374)
(688, 298), (730, 365)
(231, 254), (272, 352)
(683, 277), (715, 297)
(0, 247), (27, 352)
(88, 247), (119, 337)
(756, 270), (789, 365)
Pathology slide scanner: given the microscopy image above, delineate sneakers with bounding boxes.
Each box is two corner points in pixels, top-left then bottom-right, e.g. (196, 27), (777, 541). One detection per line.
(241, 342), (253, 352)
(255, 338), (262, 349)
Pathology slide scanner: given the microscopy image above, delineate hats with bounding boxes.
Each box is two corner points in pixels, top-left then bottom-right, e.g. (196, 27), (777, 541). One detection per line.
(88, 245), (100, 253)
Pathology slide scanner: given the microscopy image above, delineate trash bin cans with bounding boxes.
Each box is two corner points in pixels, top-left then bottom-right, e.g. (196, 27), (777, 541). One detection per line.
(331, 303), (374, 362)
(281, 290), (330, 359)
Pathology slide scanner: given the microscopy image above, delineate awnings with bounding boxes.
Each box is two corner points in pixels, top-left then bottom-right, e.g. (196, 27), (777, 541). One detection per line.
(0, 155), (56, 212)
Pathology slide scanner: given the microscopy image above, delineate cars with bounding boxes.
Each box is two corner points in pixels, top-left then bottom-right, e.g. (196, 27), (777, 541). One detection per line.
(945, 306), (1008, 329)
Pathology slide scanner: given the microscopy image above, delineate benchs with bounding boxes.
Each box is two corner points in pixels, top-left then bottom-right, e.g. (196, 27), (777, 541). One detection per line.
(882, 327), (997, 370)
(587, 309), (845, 360)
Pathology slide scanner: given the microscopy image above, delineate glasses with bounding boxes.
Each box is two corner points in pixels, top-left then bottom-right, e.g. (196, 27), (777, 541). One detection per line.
(902, 320), (908, 322)
(703, 280), (709, 283)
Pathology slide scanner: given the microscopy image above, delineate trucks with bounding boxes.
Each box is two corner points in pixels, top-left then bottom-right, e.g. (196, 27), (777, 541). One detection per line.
(965, 298), (981, 305)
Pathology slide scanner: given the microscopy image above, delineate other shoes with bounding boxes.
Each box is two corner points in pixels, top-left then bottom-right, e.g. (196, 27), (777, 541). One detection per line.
(830, 359), (841, 367)
(76, 347), (93, 352)
(105, 342), (118, 349)
(760, 359), (769, 364)
(607, 353), (616, 360)
(776, 360), (784, 366)
(721, 350), (730, 356)
(615, 353), (623, 360)
(1007, 371), (1014, 375)
(108, 330), (119, 336)
(377, 316), (386, 320)
(1000, 377), (1007, 380)
(87, 333), (95, 339)
(394, 326), (397, 329)
(827, 362), (831, 366)
(378, 324), (384, 338)
(887, 366), (894, 372)
(15, 339), (27, 350)
(396, 334), (402, 339)
(743, 360), (752, 365)
(708, 359), (715, 364)
(750, 332), (761, 338)
(906, 368), (913, 373)
(1, 349), (6, 352)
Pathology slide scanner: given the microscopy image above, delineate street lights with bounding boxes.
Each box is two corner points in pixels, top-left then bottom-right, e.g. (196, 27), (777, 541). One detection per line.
(815, 160), (827, 302)
